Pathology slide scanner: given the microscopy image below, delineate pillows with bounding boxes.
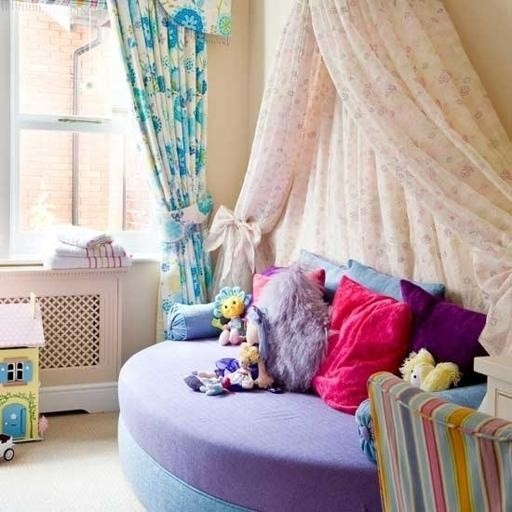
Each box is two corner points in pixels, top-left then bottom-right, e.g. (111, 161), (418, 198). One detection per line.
(400, 277), (487, 380)
(297, 251), (346, 290)
(252, 269), (326, 305)
(348, 259), (445, 303)
(314, 274), (410, 415)
(167, 301), (220, 340)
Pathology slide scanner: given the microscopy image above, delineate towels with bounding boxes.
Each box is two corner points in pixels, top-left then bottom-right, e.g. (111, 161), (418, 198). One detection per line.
(31, 223), (133, 268)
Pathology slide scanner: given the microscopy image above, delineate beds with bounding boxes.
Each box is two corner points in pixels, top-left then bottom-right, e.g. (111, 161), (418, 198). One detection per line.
(117, 254), (486, 512)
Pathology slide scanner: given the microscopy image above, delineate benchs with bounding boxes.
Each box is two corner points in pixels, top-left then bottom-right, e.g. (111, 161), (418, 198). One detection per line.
(368, 371), (511, 510)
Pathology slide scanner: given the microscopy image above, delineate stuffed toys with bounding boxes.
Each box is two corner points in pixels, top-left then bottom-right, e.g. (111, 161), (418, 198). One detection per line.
(399, 349), (460, 392)
(184, 286), (274, 396)
